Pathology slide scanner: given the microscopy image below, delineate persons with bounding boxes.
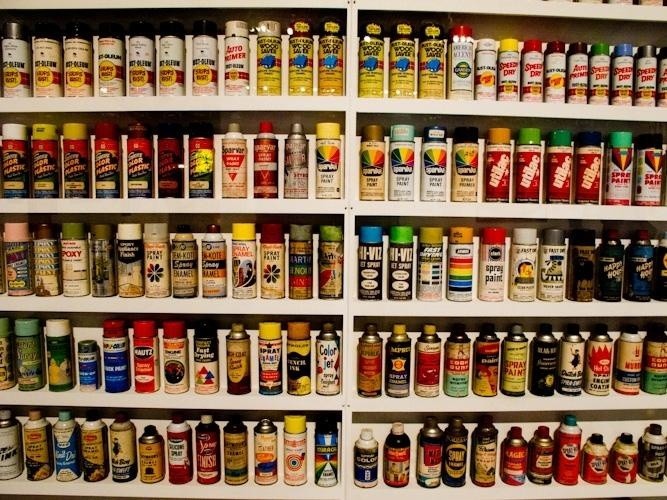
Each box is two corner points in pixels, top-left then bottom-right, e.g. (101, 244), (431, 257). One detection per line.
(244, 263), (255, 287)
(112, 438), (120, 456)
(571, 348), (580, 371)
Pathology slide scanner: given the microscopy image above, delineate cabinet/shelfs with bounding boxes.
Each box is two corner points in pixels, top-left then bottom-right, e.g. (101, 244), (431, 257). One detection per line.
(1, 0), (666, 500)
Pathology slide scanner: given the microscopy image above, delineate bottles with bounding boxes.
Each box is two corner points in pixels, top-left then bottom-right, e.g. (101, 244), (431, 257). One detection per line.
(1, 408), (665, 488)
(4, 315), (666, 402)
(4, 122), (667, 207)
(3, 220), (667, 306)
(0, 15), (666, 108)
